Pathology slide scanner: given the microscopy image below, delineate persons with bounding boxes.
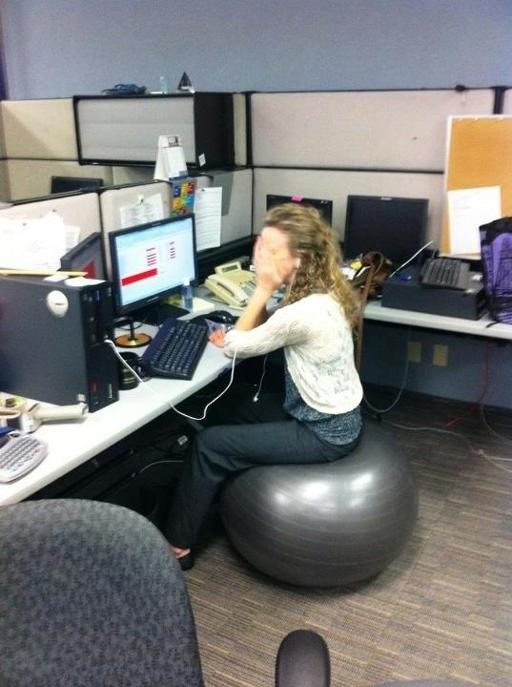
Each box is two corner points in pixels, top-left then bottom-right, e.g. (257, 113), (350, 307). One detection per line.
(146, 200), (365, 570)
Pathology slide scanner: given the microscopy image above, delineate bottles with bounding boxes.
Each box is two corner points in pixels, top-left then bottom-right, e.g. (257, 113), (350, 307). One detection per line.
(182, 277), (194, 315)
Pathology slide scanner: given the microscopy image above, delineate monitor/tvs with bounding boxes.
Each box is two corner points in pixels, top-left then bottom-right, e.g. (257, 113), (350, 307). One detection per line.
(343, 194), (429, 268)
(60, 231), (109, 283)
(266, 194), (333, 228)
(110, 212), (198, 326)
(50, 174), (104, 193)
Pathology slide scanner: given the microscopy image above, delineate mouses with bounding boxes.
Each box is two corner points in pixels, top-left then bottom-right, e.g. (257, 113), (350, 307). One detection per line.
(203, 309), (234, 324)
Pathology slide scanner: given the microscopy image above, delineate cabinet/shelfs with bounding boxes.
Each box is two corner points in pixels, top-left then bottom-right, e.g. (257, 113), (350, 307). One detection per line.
(70, 91), (236, 171)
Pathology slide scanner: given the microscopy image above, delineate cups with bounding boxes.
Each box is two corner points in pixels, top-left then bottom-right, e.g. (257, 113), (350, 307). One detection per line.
(115, 351), (151, 390)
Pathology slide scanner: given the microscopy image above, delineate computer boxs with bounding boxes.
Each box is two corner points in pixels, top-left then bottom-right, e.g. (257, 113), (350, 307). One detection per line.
(0, 265), (119, 413)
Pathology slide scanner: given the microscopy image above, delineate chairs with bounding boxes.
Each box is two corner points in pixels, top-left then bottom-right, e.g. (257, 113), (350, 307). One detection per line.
(0, 498), (331, 687)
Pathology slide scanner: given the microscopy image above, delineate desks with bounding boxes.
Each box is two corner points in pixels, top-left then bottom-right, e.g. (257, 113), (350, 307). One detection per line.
(0, 232), (512, 508)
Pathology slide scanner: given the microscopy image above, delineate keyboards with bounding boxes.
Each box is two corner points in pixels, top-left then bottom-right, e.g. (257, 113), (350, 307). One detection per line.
(139, 318), (209, 381)
(423, 258), (470, 291)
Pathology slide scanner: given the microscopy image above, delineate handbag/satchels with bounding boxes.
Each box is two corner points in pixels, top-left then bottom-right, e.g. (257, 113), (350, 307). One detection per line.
(478, 216), (512, 326)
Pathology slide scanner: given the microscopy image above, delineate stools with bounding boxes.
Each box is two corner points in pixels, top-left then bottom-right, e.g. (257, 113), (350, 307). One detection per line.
(215, 419), (416, 590)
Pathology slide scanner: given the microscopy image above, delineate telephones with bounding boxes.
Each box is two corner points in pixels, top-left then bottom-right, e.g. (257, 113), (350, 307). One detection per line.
(204, 262), (256, 307)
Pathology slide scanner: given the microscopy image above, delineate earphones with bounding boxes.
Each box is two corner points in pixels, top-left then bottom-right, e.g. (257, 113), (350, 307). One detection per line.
(252, 392), (259, 402)
(296, 256), (301, 268)
